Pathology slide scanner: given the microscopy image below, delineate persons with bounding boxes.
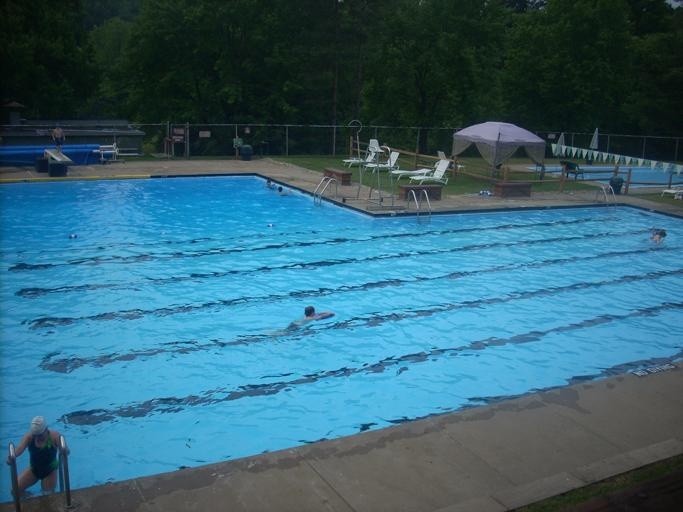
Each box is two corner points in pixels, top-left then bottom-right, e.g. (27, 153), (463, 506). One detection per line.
(51, 122), (66, 150)
(6, 416), (70, 497)
(277, 187), (285, 195)
(269, 305), (335, 335)
(266, 178), (272, 188)
(652, 231), (666, 243)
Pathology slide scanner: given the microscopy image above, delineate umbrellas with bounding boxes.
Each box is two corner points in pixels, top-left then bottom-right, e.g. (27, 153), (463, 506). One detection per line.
(552, 134), (566, 163)
(589, 128), (599, 156)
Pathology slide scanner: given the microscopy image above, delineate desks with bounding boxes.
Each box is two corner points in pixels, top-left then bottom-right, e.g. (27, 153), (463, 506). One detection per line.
(661, 189), (683, 200)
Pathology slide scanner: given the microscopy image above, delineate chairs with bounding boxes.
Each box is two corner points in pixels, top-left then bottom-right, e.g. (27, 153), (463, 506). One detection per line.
(560, 160), (585, 181)
(341, 153), (376, 169)
(361, 151), (400, 174)
(408, 159), (450, 185)
(391, 167), (432, 184)
(368, 139), (385, 160)
(437, 151), (464, 171)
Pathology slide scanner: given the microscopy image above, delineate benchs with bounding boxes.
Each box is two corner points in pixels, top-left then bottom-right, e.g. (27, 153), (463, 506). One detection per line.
(398, 184), (443, 202)
(494, 182), (532, 200)
(323, 167), (352, 187)
